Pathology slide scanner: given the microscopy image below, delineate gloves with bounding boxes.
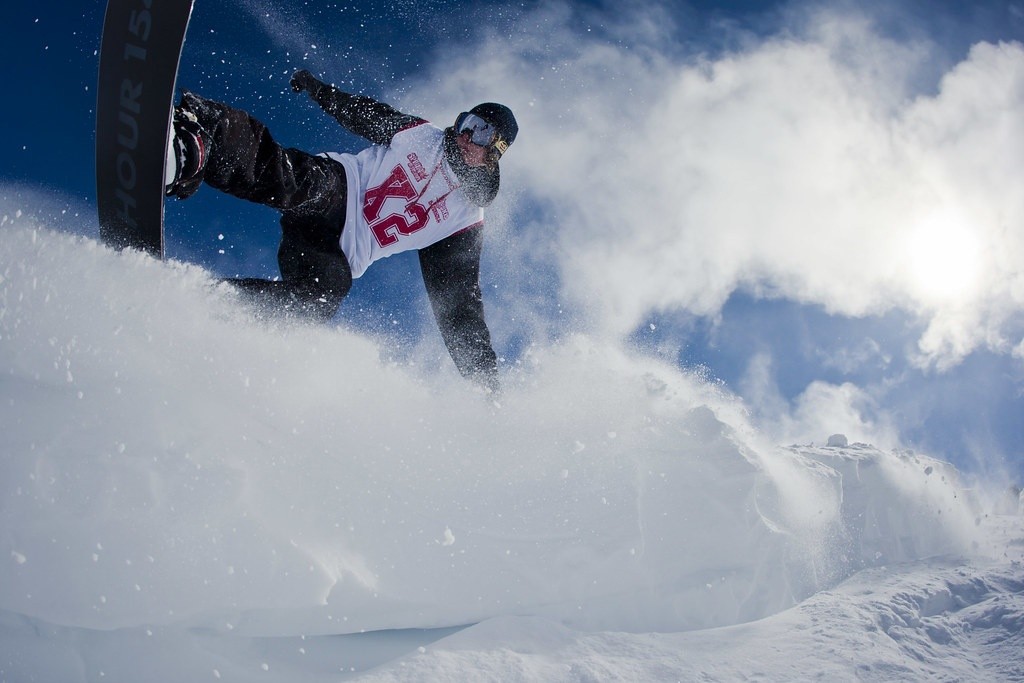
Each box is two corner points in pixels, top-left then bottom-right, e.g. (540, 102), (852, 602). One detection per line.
(472, 359), (500, 393)
(289, 69), (325, 102)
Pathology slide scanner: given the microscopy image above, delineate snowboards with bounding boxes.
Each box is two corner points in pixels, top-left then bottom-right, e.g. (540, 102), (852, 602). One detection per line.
(94, 0), (199, 263)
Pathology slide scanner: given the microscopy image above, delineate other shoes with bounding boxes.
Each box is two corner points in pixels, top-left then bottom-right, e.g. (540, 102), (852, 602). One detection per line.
(166, 104), (204, 200)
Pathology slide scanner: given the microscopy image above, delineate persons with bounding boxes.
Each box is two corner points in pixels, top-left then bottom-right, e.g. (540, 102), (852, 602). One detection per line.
(165, 69), (519, 403)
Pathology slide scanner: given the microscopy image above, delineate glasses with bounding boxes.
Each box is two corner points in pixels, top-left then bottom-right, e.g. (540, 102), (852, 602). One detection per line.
(453, 111), (501, 147)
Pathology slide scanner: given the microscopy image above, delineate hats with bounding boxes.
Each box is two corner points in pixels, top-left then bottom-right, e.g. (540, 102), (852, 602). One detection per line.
(466, 103), (518, 165)
